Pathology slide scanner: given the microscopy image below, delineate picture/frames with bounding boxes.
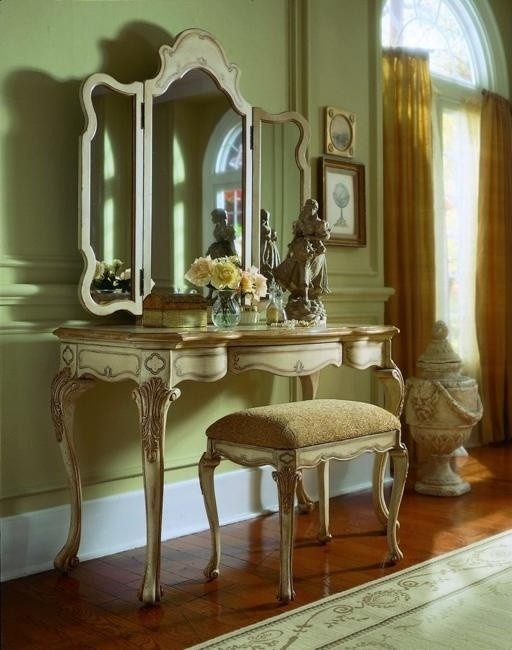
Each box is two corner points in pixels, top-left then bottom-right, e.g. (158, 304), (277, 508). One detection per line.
(319, 152), (370, 251)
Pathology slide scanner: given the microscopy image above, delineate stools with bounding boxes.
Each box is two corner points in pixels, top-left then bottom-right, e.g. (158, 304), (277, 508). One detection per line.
(197, 397), (410, 604)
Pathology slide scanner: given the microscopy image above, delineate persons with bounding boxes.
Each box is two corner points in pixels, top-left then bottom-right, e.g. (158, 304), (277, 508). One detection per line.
(271, 196), (330, 305)
(201, 205), (237, 263)
(259, 209), (287, 297)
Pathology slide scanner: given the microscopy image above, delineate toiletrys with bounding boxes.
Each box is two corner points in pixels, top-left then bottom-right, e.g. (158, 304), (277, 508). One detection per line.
(276, 298), (286, 324)
(264, 294), (279, 325)
(238, 304), (262, 325)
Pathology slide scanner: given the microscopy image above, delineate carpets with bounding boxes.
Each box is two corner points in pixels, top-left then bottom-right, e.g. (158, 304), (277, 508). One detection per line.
(183, 528), (511, 650)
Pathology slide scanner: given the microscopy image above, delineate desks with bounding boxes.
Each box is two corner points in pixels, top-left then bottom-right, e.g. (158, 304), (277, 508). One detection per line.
(48, 322), (407, 605)
